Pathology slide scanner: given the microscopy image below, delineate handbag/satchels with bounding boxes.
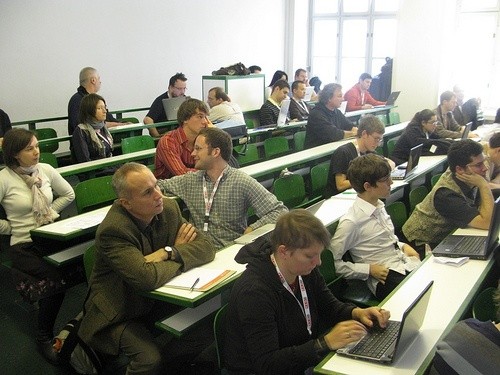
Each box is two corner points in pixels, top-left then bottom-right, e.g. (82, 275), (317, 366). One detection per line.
(222, 125), (248, 155)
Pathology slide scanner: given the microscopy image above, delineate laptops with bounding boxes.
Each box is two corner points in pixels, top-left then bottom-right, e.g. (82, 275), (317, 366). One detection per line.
(432, 197), (500, 261)
(303, 86), (315, 103)
(257, 100), (291, 128)
(161, 96), (188, 121)
(232, 199), (325, 245)
(374, 91), (401, 107)
(454, 122), (473, 141)
(338, 281), (433, 364)
(391, 144), (423, 180)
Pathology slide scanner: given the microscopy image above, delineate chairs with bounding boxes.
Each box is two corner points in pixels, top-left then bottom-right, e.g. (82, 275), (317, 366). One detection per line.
(0, 114), (446, 375)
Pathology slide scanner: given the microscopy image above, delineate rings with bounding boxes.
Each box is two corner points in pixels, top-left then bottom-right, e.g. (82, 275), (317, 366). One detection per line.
(379, 309), (383, 312)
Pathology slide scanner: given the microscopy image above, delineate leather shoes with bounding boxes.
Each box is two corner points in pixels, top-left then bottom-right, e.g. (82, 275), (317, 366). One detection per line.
(36, 331), (62, 366)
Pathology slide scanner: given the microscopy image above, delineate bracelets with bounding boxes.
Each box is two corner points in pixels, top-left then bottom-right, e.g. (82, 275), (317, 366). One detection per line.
(314, 335), (329, 356)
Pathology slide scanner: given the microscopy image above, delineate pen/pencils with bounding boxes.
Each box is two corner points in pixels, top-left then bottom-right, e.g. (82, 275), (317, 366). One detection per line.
(189, 277), (201, 292)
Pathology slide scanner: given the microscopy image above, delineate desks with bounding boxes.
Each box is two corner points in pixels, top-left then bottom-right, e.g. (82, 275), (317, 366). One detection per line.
(313, 228), (500, 374)
(29, 122), (407, 240)
(38, 120), (178, 145)
(203, 74), (265, 113)
(149, 155), (448, 310)
(57, 105), (397, 178)
(9, 107), (152, 131)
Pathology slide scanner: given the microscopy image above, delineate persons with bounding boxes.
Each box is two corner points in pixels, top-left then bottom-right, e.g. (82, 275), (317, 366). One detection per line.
(0, 128), (76, 366)
(398, 138), (495, 260)
(76, 161), (216, 375)
(343, 73), (386, 111)
(329, 153), (420, 301)
(229, 208), (390, 375)
(478, 108), (500, 189)
(156, 127), (289, 248)
(430, 278), (500, 375)
(303, 82), (359, 148)
(154, 99), (214, 180)
(249, 66), (323, 127)
(206, 86), (245, 126)
(324, 114), (395, 198)
(71, 93), (114, 181)
(396, 91), (483, 154)
(143, 72), (187, 139)
(68, 67), (130, 149)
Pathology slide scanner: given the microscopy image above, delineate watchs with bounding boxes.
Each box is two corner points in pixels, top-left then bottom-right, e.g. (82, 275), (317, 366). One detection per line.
(164, 246), (172, 260)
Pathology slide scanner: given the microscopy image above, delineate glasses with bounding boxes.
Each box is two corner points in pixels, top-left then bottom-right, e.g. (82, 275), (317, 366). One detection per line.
(361, 128), (383, 142)
(425, 121), (438, 125)
